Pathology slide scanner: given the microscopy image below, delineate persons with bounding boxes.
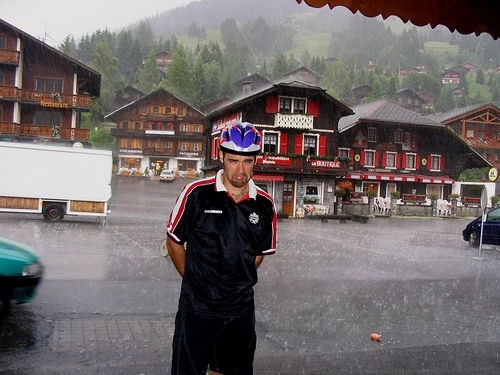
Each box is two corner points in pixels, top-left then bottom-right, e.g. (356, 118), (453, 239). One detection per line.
(166, 122), (277, 375)
(129, 162), (162, 176)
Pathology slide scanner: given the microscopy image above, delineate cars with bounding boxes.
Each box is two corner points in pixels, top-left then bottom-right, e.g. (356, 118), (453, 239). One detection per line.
(0, 238), (42, 319)
(159, 169), (176, 183)
(462, 205), (500, 249)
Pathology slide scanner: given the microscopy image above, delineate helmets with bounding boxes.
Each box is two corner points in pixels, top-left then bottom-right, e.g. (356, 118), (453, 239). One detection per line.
(218, 121), (265, 156)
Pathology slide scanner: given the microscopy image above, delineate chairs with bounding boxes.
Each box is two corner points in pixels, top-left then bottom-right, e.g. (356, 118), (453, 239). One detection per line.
(362, 196), (464, 216)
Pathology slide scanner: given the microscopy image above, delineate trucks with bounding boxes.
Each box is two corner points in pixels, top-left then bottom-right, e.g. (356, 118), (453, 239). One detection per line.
(0, 139), (113, 224)
